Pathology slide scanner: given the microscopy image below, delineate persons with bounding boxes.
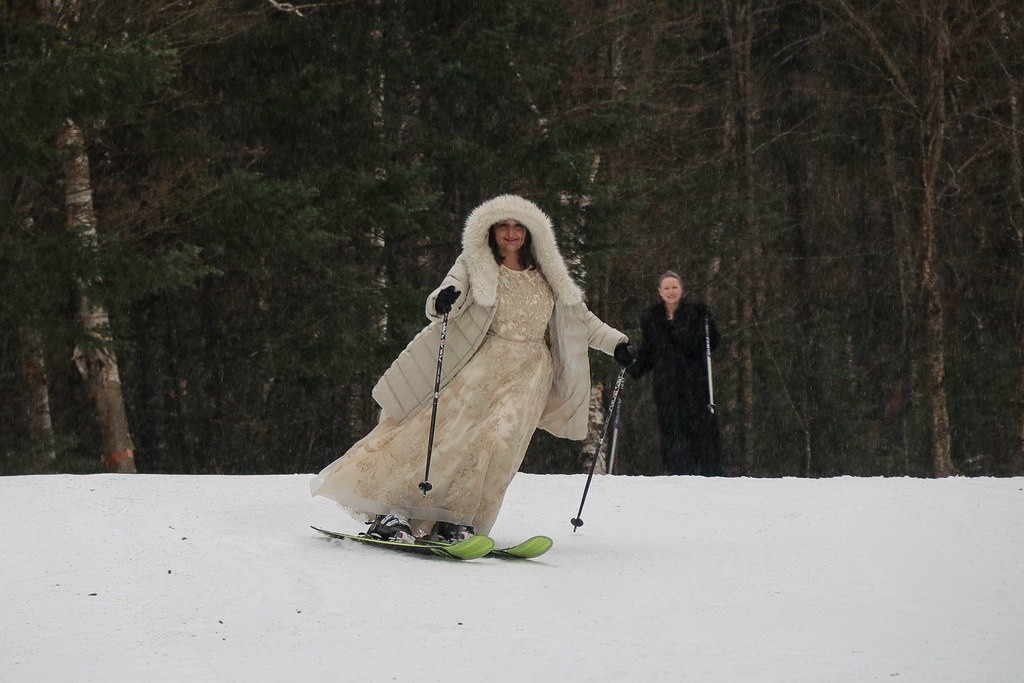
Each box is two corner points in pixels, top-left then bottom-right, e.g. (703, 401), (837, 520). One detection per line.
(310, 195), (638, 543)
(632, 272), (724, 477)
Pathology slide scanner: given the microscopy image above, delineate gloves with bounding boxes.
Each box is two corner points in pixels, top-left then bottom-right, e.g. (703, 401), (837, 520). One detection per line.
(614, 343), (638, 368)
(698, 304), (711, 317)
(626, 364), (640, 379)
(435, 286), (461, 315)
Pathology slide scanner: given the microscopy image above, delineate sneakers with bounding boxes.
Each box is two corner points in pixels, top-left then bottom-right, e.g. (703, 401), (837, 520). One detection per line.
(365, 515), (412, 540)
(430, 521), (475, 544)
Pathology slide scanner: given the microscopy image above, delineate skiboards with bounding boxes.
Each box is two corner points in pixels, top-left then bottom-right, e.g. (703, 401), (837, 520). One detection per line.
(308, 525), (554, 562)
